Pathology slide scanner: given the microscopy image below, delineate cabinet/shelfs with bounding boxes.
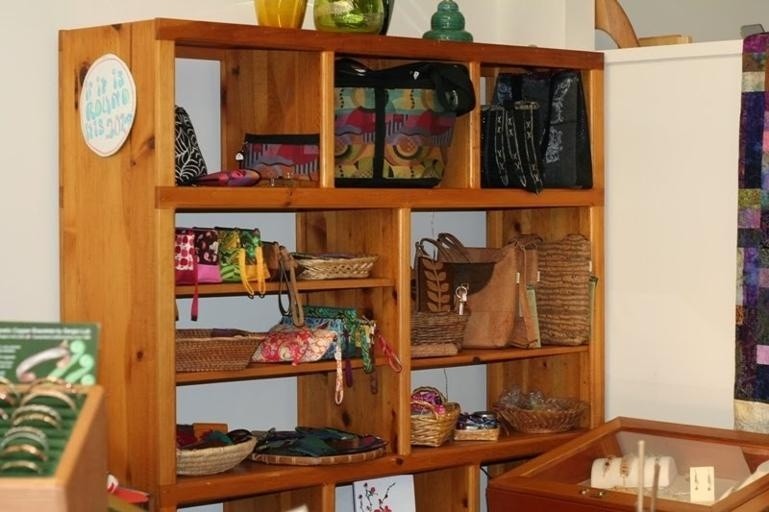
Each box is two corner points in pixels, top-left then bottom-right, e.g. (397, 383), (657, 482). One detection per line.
(484, 416), (768, 512)
(57, 15), (608, 512)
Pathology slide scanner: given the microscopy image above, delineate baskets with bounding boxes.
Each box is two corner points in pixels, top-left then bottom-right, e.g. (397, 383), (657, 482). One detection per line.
(172, 327), (270, 372)
(287, 250), (379, 282)
(407, 242), (470, 359)
(174, 432), (258, 478)
(493, 396), (590, 435)
(408, 387), (462, 448)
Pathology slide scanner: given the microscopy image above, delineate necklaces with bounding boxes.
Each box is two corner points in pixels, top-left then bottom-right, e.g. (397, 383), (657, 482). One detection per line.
(601, 450), (713, 502)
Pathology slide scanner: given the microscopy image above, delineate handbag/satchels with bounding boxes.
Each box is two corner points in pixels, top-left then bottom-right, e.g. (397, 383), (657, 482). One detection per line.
(252, 304), (402, 405)
(173, 104), (322, 190)
(175, 226), (290, 322)
(480, 65), (593, 192)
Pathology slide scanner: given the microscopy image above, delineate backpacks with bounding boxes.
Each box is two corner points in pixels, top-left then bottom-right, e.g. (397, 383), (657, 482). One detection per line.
(416, 228), (592, 350)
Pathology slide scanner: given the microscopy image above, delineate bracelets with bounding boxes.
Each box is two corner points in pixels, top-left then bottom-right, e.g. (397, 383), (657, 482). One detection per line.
(1, 372), (78, 476)
